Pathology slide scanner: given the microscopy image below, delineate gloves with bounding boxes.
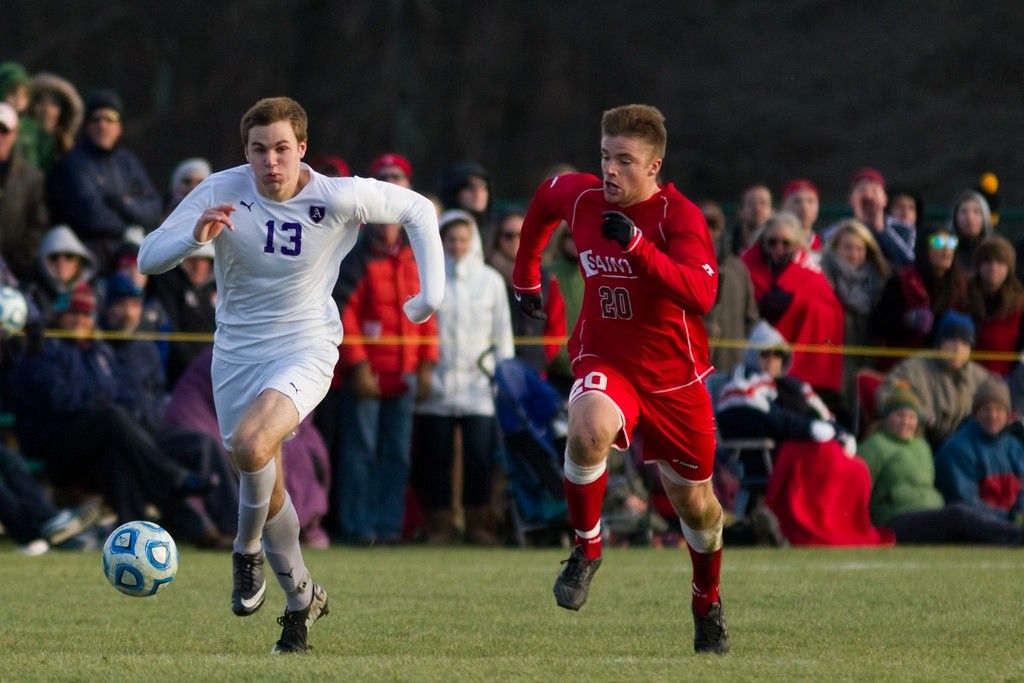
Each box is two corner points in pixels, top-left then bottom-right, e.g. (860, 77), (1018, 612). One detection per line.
(840, 431), (857, 455)
(810, 422), (835, 441)
(600, 210), (636, 248)
(515, 291), (548, 319)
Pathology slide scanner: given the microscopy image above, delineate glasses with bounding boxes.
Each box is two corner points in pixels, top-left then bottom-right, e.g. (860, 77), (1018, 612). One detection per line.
(49, 252), (75, 262)
(928, 236), (958, 249)
(499, 230), (522, 240)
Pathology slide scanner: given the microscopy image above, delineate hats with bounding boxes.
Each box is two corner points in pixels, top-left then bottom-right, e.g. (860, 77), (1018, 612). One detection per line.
(105, 272), (146, 304)
(370, 153), (409, 177)
(0, 101), (18, 131)
(327, 158), (350, 178)
(937, 310), (974, 344)
(974, 374), (1010, 416)
(785, 177), (819, 199)
(52, 282), (98, 317)
(884, 378), (920, 417)
(848, 168), (885, 189)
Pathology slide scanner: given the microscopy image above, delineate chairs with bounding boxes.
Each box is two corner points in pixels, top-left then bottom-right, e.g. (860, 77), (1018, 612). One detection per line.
(705, 373), (776, 487)
(855, 369), (886, 437)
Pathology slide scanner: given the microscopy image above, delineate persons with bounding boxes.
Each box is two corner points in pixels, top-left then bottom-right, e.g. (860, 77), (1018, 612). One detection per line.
(137, 96), (446, 648)
(0, 61), (585, 553)
(513, 105), (732, 653)
(604, 167), (1024, 546)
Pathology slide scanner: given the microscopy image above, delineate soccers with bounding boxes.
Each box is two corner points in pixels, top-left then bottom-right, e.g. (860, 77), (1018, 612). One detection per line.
(0, 284), (29, 339)
(101, 520), (179, 597)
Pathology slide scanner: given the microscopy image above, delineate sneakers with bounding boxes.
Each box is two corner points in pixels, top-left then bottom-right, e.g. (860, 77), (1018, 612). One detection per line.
(232, 546), (267, 617)
(554, 545), (602, 610)
(272, 582), (329, 653)
(691, 594), (730, 654)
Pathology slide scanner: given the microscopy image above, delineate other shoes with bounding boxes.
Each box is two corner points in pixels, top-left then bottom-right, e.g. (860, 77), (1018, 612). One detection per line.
(27, 491), (792, 556)
